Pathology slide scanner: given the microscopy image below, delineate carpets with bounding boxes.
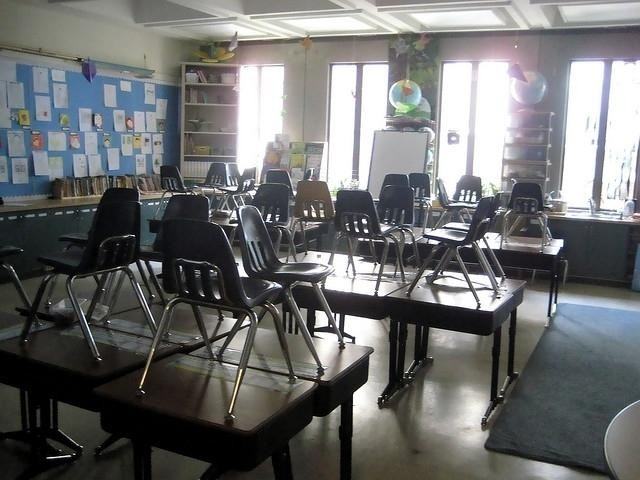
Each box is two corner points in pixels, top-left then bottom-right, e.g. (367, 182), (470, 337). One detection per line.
(483, 301), (640, 474)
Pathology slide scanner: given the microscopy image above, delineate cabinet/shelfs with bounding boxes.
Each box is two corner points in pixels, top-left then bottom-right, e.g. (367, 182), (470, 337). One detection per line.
(500, 109), (556, 196)
(179, 61), (242, 181)
(0, 187), (256, 287)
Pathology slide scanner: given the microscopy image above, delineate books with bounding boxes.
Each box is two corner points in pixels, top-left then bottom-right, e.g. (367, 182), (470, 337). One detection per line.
(198, 71), (207, 83)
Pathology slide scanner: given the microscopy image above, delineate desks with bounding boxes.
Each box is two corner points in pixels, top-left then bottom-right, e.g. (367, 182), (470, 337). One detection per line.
(189, 326), (375, 480)
(92, 352), (318, 480)
(0, 324), (183, 456)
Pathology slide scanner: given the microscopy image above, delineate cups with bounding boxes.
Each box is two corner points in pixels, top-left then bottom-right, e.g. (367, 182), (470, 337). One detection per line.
(622, 197), (635, 216)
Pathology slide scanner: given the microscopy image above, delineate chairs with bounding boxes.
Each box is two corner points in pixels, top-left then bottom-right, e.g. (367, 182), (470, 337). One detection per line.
(604, 399), (640, 479)
